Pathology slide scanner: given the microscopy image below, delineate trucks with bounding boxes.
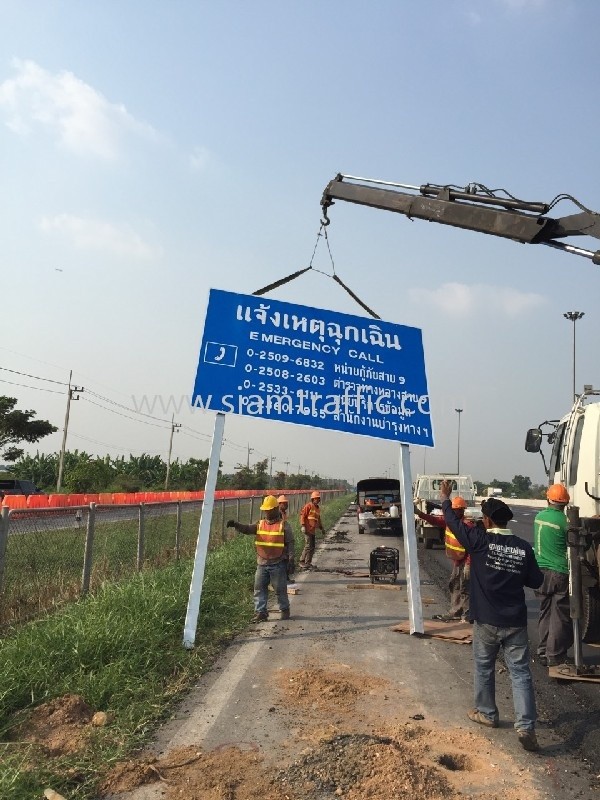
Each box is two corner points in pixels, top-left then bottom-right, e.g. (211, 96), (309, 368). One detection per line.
(411, 473), (485, 548)
(354, 478), (403, 535)
(523, 386), (600, 677)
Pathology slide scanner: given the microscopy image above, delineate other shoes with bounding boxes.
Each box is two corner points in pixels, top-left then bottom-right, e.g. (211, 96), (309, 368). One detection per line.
(288, 579), (295, 583)
(299, 562), (307, 568)
(539, 658), (546, 666)
(267, 584), (274, 592)
(439, 612), (462, 620)
(306, 563), (313, 570)
(464, 617), (475, 624)
(548, 657), (570, 666)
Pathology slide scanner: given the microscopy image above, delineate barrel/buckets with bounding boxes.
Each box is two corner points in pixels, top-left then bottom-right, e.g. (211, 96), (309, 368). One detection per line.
(390, 506), (398, 518)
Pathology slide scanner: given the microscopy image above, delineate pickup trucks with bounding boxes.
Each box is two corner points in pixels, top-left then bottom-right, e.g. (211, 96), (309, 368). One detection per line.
(0, 479), (50, 499)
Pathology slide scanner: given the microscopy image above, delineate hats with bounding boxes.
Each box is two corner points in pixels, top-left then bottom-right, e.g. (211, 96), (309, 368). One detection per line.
(482, 499), (513, 526)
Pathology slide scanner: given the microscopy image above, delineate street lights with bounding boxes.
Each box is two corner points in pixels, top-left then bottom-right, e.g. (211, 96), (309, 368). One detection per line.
(454, 408), (464, 474)
(563, 311), (586, 403)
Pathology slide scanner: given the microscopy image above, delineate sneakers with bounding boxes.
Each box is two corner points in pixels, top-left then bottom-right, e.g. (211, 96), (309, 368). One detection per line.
(250, 613), (267, 623)
(280, 609), (290, 620)
(517, 729), (539, 751)
(467, 708), (499, 728)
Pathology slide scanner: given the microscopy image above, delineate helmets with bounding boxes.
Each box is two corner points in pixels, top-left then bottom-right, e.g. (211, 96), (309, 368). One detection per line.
(546, 484), (570, 502)
(451, 496), (467, 509)
(311, 491), (320, 498)
(260, 495), (279, 510)
(277, 495), (288, 502)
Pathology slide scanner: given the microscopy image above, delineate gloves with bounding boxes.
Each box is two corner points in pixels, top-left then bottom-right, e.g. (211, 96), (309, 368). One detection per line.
(226, 520), (234, 528)
(301, 527), (306, 533)
(287, 562), (295, 574)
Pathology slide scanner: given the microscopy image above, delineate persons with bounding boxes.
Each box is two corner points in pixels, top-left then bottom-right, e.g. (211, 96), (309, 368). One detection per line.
(440, 479), (540, 753)
(414, 497), (474, 620)
(227, 494), (296, 620)
(534, 484), (574, 664)
(298, 491), (322, 568)
